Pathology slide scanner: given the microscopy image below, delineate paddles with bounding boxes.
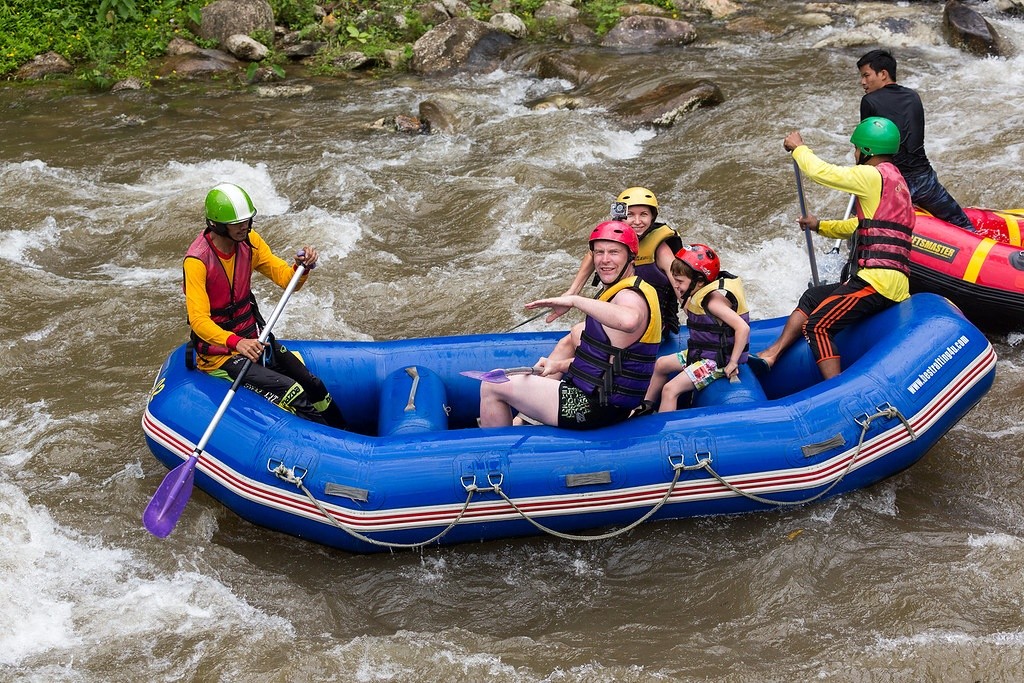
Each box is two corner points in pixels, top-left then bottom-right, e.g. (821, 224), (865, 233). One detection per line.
(142, 248), (318, 540)
(786, 133), (827, 289)
(822, 192), (857, 255)
(460, 366), (546, 385)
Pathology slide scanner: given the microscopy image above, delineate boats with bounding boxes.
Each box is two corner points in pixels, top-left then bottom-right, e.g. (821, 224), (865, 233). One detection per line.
(139, 292), (999, 558)
(908, 200), (1023, 341)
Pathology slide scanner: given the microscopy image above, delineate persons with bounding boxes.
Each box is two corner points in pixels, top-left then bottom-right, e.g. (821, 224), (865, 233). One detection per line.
(627, 242), (751, 417)
(856, 50), (978, 233)
(478, 187), (684, 430)
(184, 183), (350, 432)
(748, 117), (915, 382)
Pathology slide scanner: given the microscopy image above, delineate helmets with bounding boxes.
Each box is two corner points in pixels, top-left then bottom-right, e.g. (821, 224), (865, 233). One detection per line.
(616, 187), (658, 217)
(205, 184), (257, 235)
(850, 116), (901, 156)
(675, 243), (720, 283)
(589, 221), (639, 256)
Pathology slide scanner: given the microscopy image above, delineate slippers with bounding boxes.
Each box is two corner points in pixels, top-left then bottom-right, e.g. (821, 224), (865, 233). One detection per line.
(746, 353), (770, 374)
